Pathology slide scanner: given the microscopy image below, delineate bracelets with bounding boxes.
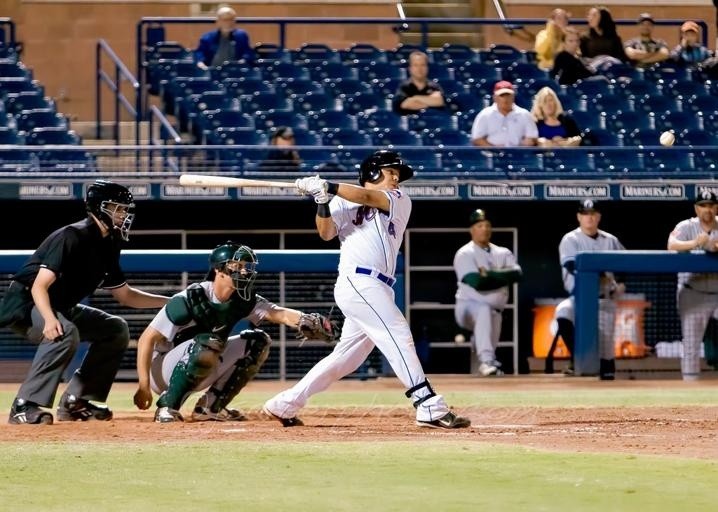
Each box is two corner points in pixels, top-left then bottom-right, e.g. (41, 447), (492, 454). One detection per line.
(316, 203), (332, 218)
(327, 181), (339, 195)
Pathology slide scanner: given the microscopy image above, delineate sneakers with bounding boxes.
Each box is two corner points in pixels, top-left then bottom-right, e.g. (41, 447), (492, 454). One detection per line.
(56, 391), (113, 423)
(415, 409), (471, 430)
(7, 396), (53, 426)
(479, 360), (502, 377)
(262, 402), (305, 427)
(192, 396), (245, 421)
(152, 405), (184, 423)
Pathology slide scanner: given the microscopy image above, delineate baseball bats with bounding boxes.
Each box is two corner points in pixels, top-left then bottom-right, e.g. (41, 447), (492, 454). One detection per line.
(179, 174), (330, 192)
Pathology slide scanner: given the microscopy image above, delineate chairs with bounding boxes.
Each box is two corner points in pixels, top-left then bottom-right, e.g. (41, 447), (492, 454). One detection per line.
(0, 39), (99, 197)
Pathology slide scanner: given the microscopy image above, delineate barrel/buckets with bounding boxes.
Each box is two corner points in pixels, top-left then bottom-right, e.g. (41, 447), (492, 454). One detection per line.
(610, 293), (653, 360)
(532, 296), (573, 359)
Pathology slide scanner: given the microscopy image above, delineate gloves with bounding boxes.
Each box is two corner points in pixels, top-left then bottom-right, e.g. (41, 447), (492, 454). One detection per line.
(293, 174), (330, 204)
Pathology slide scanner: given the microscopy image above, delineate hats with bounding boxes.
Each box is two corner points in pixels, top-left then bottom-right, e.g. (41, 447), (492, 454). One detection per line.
(638, 12), (655, 25)
(694, 190), (718, 205)
(465, 207), (495, 229)
(493, 79), (516, 97)
(273, 126), (296, 140)
(680, 20), (700, 34)
(576, 198), (601, 213)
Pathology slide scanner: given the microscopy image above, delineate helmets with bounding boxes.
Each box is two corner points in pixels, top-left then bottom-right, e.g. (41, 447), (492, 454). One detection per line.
(85, 178), (137, 208)
(358, 150), (413, 188)
(207, 240), (257, 268)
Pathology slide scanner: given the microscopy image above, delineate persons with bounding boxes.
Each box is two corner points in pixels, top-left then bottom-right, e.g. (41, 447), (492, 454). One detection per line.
(132, 243), (345, 425)
(620, 13), (673, 66)
(390, 48), (448, 113)
(469, 80), (541, 148)
(261, 149), (472, 430)
(194, 5), (255, 73)
(577, 5), (633, 62)
(667, 190), (717, 380)
(666, 19), (717, 69)
(530, 9), (583, 74)
(258, 127), (302, 167)
(2, 178), (176, 425)
(555, 196), (626, 382)
(548, 27), (596, 87)
(527, 86), (587, 149)
(452, 207), (524, 378)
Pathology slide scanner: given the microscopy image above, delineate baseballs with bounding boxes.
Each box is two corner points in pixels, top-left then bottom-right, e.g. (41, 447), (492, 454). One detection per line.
(659, 131), (675, 147)
(454, 334), (465, 343)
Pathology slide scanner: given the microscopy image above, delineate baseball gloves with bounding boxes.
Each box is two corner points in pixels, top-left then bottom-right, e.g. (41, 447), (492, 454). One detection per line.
(299, 312), (337, 343)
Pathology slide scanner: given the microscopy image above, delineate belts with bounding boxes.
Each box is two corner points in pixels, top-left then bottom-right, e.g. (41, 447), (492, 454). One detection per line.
(355, 264), (398, 289)
(684, 283), (718, 295)
